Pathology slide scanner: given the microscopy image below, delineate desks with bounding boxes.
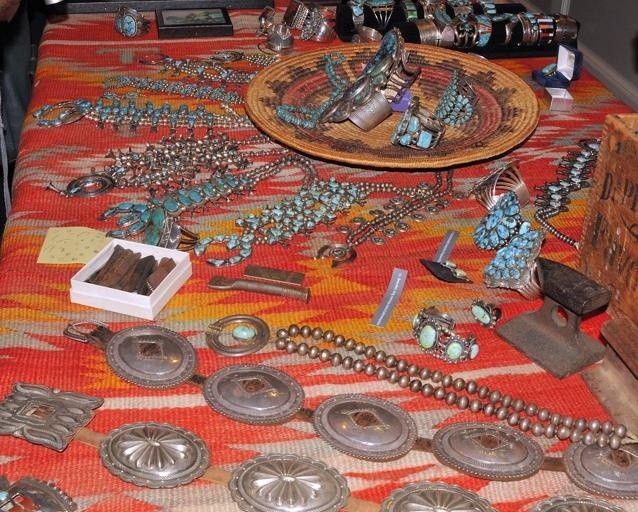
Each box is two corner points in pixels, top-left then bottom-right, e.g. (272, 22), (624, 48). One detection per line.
(1, 0), (637, 511)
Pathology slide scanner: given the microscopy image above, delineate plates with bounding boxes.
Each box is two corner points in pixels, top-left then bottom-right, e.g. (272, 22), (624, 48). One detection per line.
(244, 41), (541, 169)
